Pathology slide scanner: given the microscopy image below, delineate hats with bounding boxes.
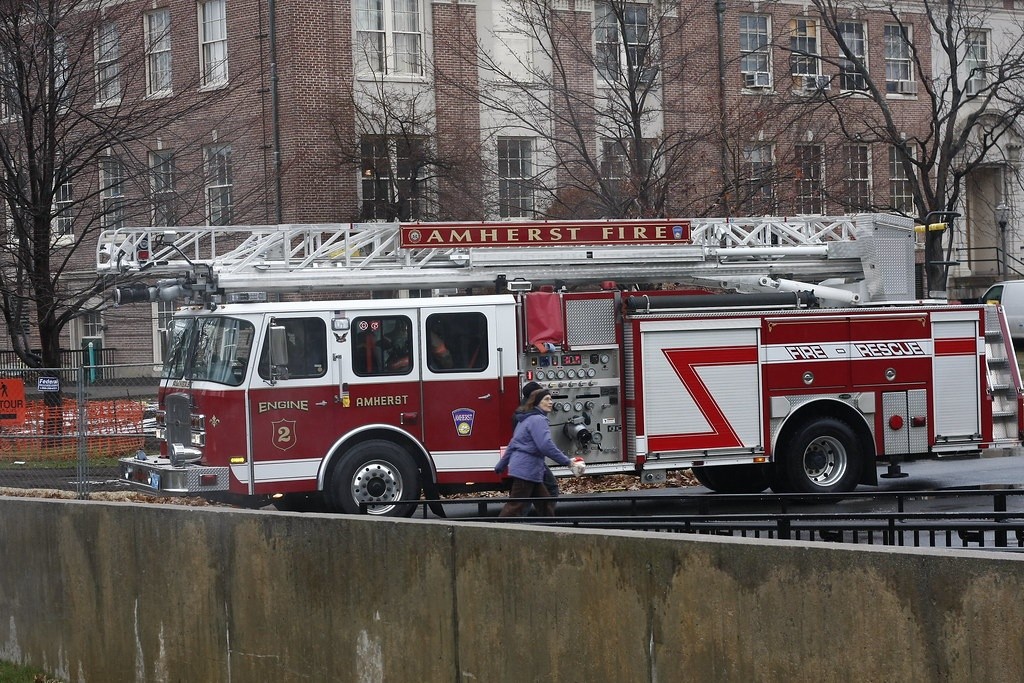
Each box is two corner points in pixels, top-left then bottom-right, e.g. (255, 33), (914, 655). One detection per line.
(522, 381), (542, 398)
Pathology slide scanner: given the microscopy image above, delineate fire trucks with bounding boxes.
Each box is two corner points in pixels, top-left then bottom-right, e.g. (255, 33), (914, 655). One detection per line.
(96, 211), (1024, 519)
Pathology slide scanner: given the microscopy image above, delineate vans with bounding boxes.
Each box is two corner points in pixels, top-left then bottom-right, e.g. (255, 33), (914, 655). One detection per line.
(976, 279), (1024, 340)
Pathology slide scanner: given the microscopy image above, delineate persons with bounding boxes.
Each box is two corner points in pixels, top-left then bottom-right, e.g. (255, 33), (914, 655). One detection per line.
(494, 382), (585, 523)
(377, 316), (452, 371)
